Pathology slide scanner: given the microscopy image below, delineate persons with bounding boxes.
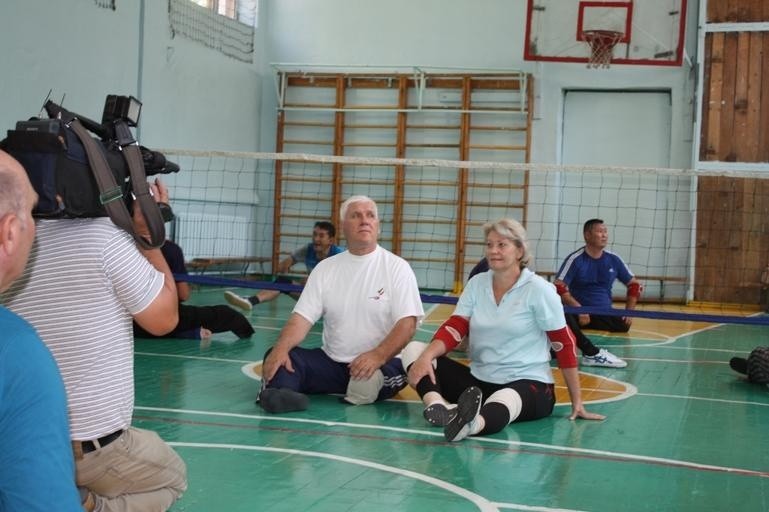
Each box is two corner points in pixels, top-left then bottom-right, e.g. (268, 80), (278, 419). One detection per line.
(0, 116), (188, 512)
(254, 195), (426, 413)
(4, 150), (89, 512)
(124, 181), (255, 342)
(451, 257), (628, 369)
(404, 216), (606, 442)
(223, 217), (352, 314)
(553, 219), (644, 332)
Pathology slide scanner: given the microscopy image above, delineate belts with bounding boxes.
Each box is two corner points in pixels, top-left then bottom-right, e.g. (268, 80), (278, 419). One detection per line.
(83, 429), (123, 452)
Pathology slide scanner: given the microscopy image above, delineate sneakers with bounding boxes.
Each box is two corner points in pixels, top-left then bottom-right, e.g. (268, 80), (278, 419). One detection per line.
(581, 348), (627, 368)
(422, 386), (483, 442)
(223, 290), (253, 310)
